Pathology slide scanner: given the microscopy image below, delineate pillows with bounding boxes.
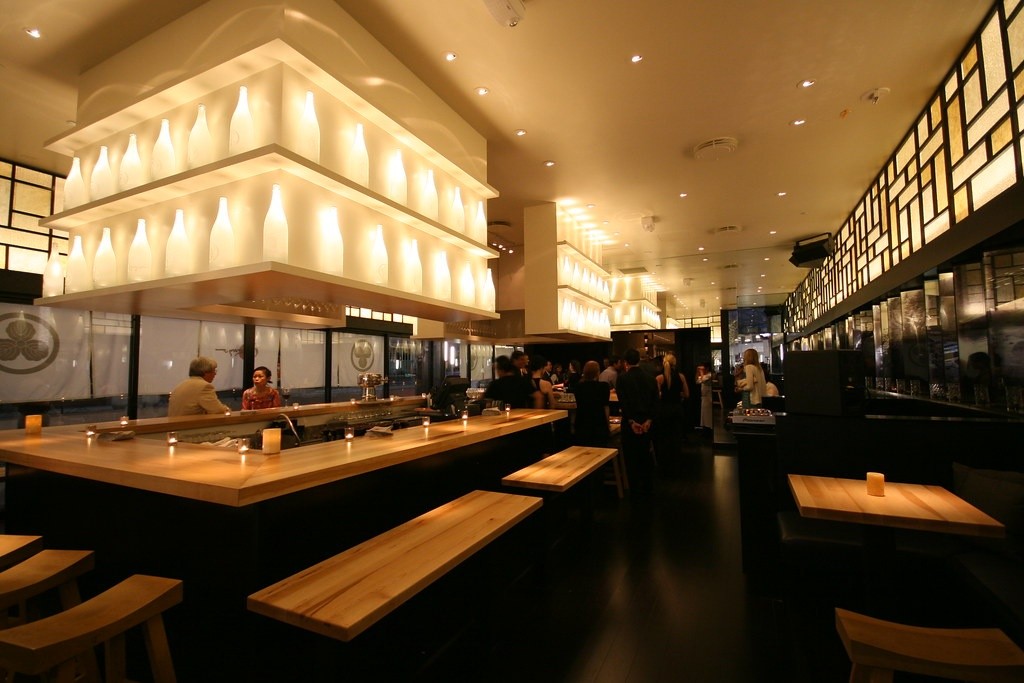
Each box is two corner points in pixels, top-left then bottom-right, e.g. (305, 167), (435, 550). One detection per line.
(952, 461), (1024, 561)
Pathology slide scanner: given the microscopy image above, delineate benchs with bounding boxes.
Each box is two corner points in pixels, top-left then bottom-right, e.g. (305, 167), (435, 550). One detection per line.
(242, 489), (545, 683)
(778, 510), (1024, 650)
(501, 444), (620, 588)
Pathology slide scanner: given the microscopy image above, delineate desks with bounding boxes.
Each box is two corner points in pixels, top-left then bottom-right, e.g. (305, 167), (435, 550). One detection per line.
(786, 470), (1007, 623)
(712, 384), (726, 408)
(0, 394), (582, 683)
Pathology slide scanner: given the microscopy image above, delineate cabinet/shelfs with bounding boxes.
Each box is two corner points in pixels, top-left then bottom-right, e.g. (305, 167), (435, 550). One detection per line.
(35, 32), (614, 345)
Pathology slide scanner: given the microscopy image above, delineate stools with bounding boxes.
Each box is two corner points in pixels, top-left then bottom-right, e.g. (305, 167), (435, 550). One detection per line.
(603, 442), (629, 498)
(833, 606), (1024, 683)
(712, 390), (724, 409)
(0, 535), (184, 683)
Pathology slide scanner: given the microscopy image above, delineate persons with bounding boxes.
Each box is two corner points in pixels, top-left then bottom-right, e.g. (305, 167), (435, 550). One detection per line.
(471, 349), (777, 489)
(242, 366), (281, 410)
(168, 356), (231, 416)
